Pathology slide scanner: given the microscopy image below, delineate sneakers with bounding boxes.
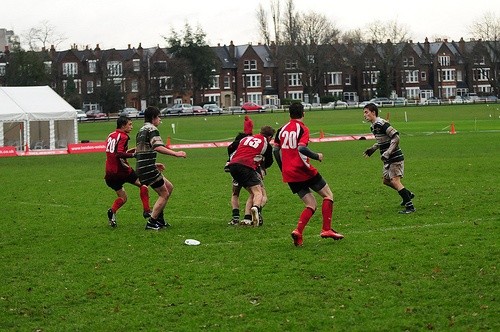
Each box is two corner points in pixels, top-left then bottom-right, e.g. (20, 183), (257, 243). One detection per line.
(398, 208), (416, 214)
(228, 216), (240, 225)
(320, 228), (344, 240)
(143, 210), (153, 219)
(145, 219), (171, 231)
(108, 209), (118, 228)
(250, 207), (263, 227)
(400, 193), (415, 206)
(241, 220), (253, 226)
(291, 228), (303, 247)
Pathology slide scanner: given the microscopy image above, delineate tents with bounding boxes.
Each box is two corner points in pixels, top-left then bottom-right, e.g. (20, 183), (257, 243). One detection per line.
(0, 85), (79, 156)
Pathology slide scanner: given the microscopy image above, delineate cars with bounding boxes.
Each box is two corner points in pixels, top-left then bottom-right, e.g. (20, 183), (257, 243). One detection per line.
(326, 96), (405, 107)
(263, 104), (278, 109)
(118, 108), (139, 117)
(449, 95), (497, 104)
(139, 108), (146, 115)
(192, 106), (208, 115)
(86, 110), (107, 118)
(300, 102), (321, 108)
(425, 96), (441, 105)
(76, 110), (87, 119)
(241, 102), (266, 114)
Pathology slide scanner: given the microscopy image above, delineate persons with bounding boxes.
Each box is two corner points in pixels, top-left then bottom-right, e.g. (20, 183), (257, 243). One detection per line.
(273, 102), (344, 247)
(228, 126), (276, 227)
(361, 103), (415, 214)
(135, 106), (186, 230)
(104, 116), (153, 227)
(224, 133), (268, 226)
(243, 115), (254, 136)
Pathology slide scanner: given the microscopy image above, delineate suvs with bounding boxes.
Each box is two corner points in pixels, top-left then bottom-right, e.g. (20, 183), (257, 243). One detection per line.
(166, 104), (193, 114)
(203, 104), (223, 115)
